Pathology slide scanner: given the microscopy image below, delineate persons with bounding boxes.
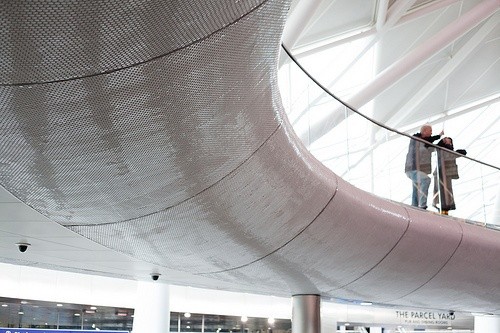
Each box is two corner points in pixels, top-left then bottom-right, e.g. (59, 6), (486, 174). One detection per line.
(433, 136), (467, 214)
(405, 123), (444, 209)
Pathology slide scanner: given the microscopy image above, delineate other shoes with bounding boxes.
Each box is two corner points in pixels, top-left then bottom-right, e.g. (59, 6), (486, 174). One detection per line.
(440, 210), (448, 215)
